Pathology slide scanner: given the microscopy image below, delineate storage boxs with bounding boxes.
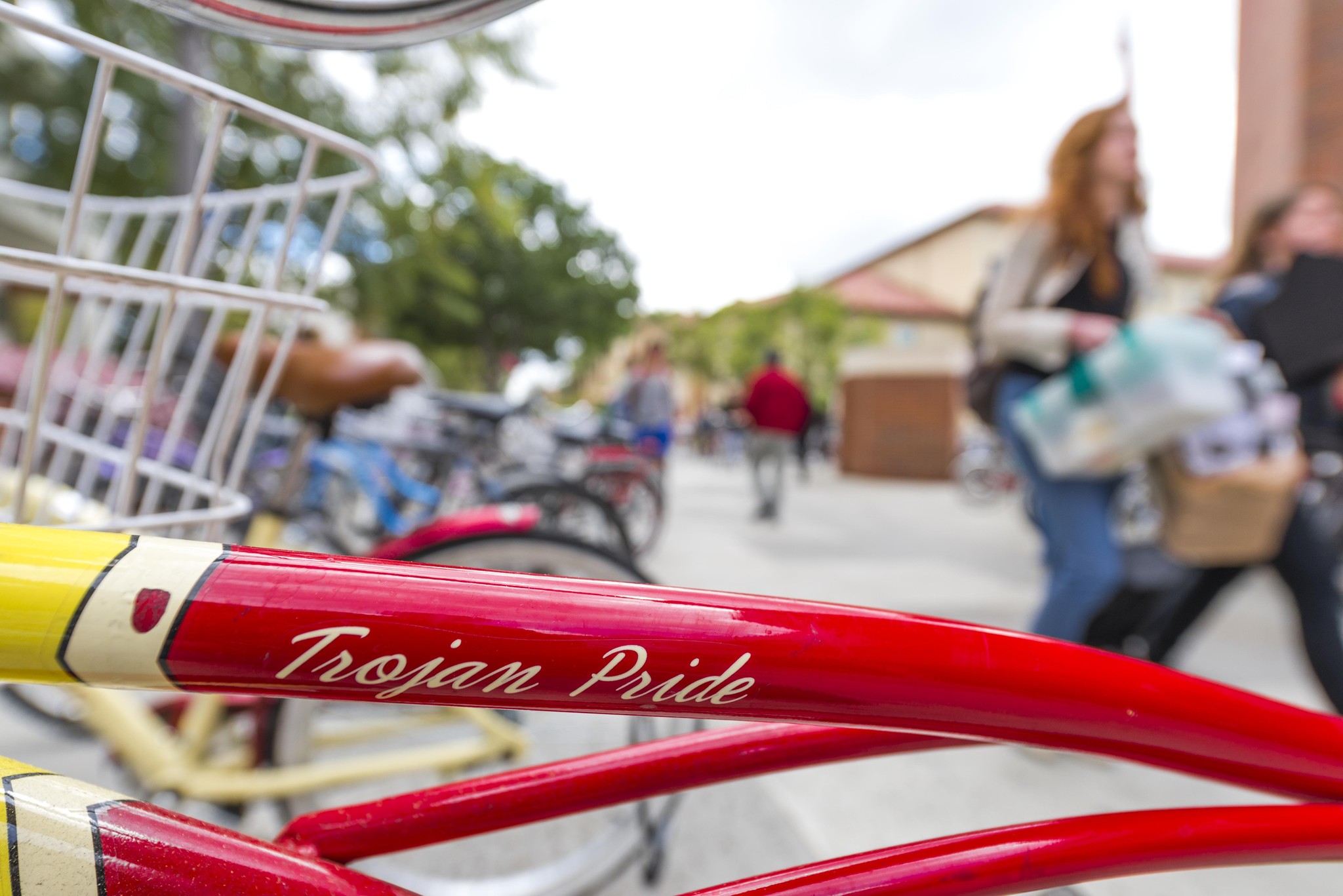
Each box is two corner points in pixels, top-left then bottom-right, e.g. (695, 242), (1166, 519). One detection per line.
(1010, 311), (1242, 482)
(1146, 311), (1307, 567)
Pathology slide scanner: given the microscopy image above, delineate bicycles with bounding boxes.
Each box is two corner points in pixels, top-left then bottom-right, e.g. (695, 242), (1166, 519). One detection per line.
(0, 325), (702, 896)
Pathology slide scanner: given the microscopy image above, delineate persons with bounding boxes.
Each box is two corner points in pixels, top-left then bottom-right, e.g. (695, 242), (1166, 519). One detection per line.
(620, 341), (677, 461)
(1125, 179), (1343, 718)
(981, 108), (1156, 647)
(743, 349), (808, 522)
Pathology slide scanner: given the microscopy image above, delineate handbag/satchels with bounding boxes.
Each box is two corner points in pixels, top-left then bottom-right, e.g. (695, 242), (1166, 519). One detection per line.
(962, 283), (1006, 428)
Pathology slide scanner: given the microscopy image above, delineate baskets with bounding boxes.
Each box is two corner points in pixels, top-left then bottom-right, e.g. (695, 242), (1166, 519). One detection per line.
(0, 0), (388, 546)
